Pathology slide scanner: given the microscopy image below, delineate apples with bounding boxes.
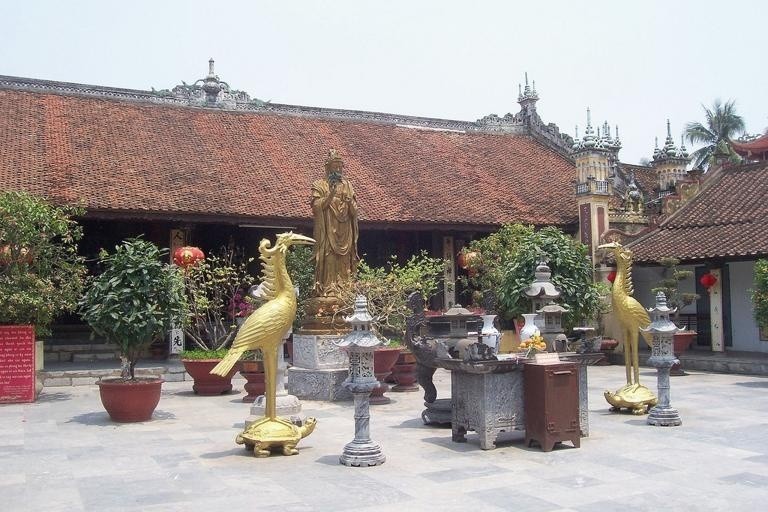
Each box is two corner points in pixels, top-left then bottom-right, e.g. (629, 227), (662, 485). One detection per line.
(520, 337), (545, 349)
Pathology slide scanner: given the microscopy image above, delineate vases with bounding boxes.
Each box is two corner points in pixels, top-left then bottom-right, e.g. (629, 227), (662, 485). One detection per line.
(601, 338), (618, 350)
(481, 313), (540, 357)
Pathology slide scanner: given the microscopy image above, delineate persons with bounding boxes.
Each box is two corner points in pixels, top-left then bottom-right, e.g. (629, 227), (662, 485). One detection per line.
(310, 150), (360, 296)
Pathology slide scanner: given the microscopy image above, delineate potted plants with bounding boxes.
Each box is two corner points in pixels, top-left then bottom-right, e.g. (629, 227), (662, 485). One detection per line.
(641, 256), (702, 375)
(77, 232), (266, 422)
(354, 250), (460, 404)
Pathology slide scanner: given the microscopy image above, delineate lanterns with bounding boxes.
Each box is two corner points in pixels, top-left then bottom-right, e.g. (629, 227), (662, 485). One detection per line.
(457, 249), (483, 278)
(607, 271), (617, 285)
(698, 271), (717, 294)
(172, 245), (206, 275)
(1, 240), (33, 270)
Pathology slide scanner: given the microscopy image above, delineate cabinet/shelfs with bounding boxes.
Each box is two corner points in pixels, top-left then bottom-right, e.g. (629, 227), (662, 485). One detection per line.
(525, 360), (580, 451)
